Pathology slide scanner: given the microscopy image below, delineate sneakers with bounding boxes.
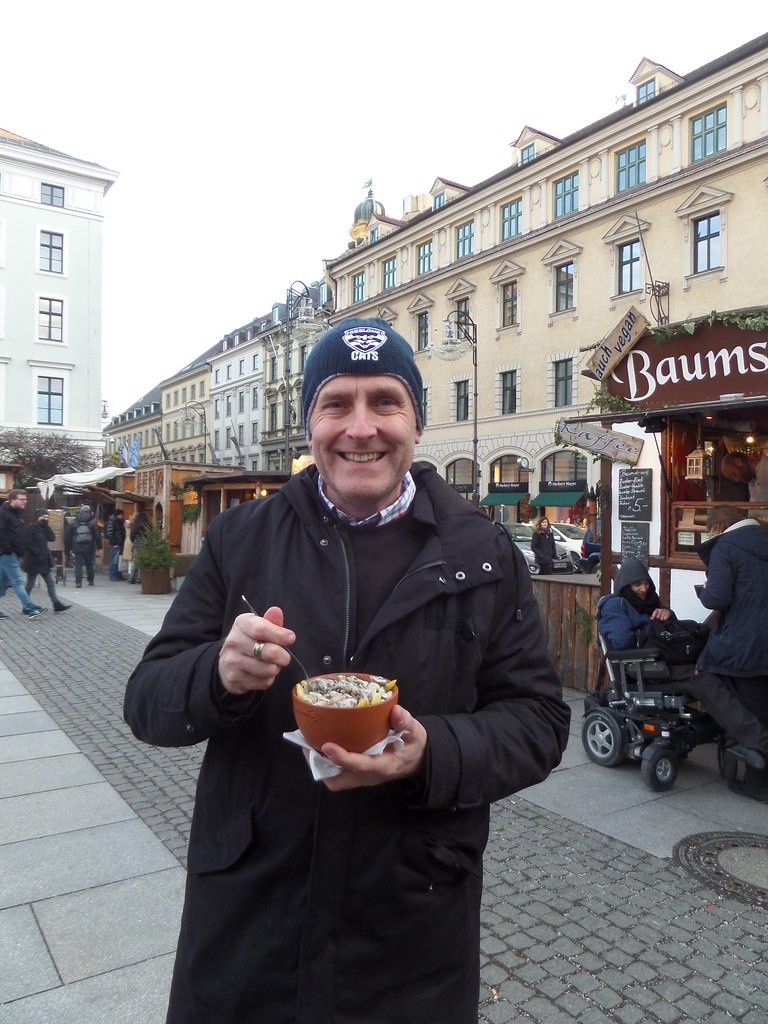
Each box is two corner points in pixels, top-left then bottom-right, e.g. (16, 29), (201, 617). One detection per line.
(0, 612), (8, 619)
(28, 607), (48, 619)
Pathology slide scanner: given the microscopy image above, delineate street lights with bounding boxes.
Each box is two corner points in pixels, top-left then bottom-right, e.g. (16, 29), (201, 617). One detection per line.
(430, 310), (480, 506)
(179, 400), (206, 463)
(285, 280), (324, 471)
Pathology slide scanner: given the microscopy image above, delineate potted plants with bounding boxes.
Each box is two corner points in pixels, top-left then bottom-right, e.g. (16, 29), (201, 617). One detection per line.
(133, 521), (178, 594)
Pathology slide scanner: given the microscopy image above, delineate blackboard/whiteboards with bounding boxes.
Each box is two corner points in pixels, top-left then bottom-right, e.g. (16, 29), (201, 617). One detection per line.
(620, 521), (649, 569)
(619, 468), (652, 521)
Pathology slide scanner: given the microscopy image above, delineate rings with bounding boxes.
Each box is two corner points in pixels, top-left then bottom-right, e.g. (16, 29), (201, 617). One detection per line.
(253, 640), (263, 662)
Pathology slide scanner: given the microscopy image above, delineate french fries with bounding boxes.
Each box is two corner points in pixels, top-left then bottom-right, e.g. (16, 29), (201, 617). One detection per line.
(295, 677), (397, 707)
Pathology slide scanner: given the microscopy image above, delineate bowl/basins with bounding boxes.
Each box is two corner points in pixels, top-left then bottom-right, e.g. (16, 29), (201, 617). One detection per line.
(292, 673), (398, 753)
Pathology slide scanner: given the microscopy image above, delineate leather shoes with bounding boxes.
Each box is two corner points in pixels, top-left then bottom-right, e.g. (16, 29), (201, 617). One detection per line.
(728, 779), (768, 804)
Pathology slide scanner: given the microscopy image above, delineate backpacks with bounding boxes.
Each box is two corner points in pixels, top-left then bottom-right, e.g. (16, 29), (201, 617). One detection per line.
(75, 520), (93, 544)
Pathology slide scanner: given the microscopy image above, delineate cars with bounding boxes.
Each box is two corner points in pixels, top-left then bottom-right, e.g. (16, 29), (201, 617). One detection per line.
(499, 523), (575, 574)
(580, 519), (602, 575)
(523, 523), (587, 574)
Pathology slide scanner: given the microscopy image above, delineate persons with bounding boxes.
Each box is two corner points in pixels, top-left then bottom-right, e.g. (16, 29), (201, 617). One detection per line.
(720, 451), (767, 523)
(689, 505), (768, 807)
(0, 488), (46, 621)
(596, 555), (768, 755)
(105, 507), (126, 581)
(123, 318), (574, 1024)
(65, 503), (102, 589)
(531, 516), (558, 574)
(129, 510), (152, 586)
(22, 508), (72, 615)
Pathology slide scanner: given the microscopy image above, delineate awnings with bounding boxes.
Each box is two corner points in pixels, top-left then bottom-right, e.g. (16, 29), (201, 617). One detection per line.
(481, 491), (530, 509)
(110, 490), (156, 503)
(530, 489), (584, 507)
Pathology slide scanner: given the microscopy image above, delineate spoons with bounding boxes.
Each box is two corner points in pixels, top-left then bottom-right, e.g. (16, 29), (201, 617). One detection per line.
(242, 594), (314, 692)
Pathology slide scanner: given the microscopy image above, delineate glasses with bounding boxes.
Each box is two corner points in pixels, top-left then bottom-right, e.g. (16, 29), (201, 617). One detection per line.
(18, 498), (27, 501)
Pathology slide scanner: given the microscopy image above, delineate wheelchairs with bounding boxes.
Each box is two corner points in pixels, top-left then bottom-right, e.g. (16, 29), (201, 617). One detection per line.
(581, 596), (738, 793)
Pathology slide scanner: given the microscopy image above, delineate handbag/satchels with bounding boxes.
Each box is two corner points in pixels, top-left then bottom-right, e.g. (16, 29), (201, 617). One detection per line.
(47, 552), (54, 568)
(659, 620), (710, 660)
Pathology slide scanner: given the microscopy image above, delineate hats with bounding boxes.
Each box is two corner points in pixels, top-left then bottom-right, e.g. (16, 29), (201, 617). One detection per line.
(34, 509), (49, 519)
(302, 317), (423, 441)
(114, 509), (124, 517)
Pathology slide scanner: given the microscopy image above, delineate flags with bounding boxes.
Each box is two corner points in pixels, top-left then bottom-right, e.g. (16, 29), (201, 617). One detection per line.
(362, 178), (372, 188)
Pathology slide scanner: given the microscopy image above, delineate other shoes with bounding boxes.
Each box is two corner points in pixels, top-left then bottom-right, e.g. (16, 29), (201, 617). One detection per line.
(76, 583), (81, 588)
(127, 577), (135, 584)
(23, 606), (40, 614)
(55, 602), (71, 611)
(110, 575), (120, 581)
(89, 581), (94, 586)
(137, 578), (141, 583)
